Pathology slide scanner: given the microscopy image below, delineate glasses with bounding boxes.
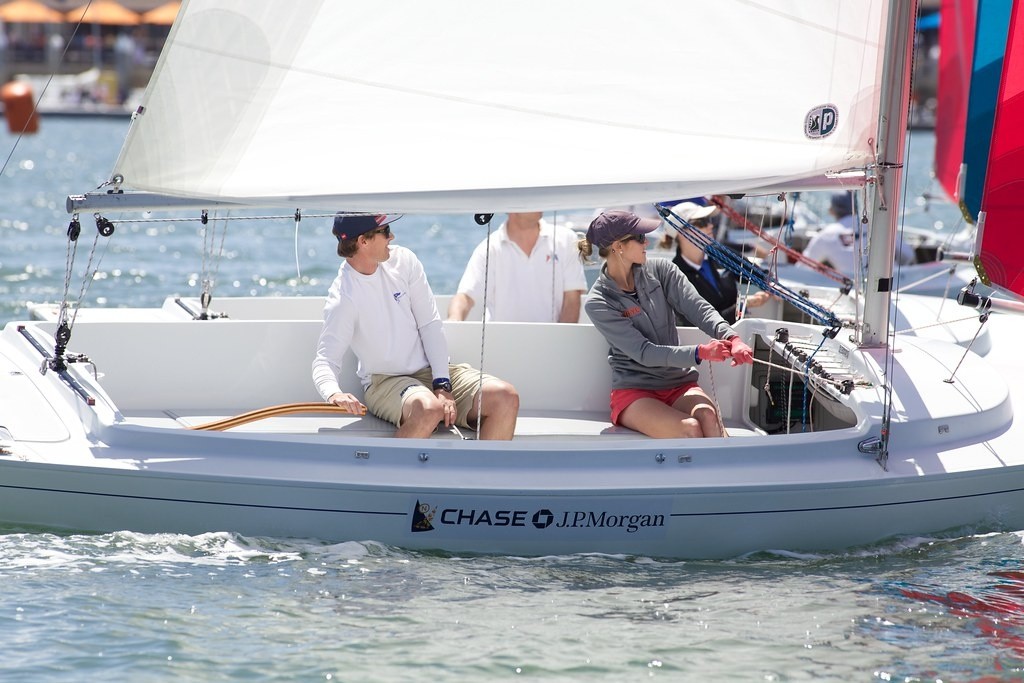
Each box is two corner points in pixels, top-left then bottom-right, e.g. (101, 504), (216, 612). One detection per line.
(683, 215), (712, 231)
(611, 233), (646, 253)
(374, 225), (390, 239)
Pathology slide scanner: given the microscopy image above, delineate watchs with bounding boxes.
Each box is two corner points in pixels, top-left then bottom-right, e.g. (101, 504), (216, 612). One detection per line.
(433, 381), (453, 393)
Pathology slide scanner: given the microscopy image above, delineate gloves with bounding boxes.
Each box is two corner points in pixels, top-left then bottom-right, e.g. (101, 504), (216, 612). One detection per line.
(698, 339), (732, 361)
(728, 335), (753, 367)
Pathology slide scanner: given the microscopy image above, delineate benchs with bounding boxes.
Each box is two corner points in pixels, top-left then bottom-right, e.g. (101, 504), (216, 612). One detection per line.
(168, 322), (768, 437)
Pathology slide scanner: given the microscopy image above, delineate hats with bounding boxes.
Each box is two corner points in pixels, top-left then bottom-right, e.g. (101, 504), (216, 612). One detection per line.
(332, 211), (403, 242)
(831, 190), (857, 215)
(587, 211), (661, 250)
(664, 201), (717, 239)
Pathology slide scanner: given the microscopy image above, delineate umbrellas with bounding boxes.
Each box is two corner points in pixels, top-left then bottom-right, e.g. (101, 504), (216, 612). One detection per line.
(65, 0), (139, 64)
(0, 0), (65, 54)
(139, 0), (183, 54)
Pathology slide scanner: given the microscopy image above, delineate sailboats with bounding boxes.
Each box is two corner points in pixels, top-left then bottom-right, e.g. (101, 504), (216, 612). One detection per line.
(0, 0), (1024, 561)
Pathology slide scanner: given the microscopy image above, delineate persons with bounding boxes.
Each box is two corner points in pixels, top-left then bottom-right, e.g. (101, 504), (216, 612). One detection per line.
(445, 213), (588, 324)
(660, 201), (773, 328)
(313, 212), (519, 440)
(577, 212), (754, 439)
(795, 188), (916, 281)
